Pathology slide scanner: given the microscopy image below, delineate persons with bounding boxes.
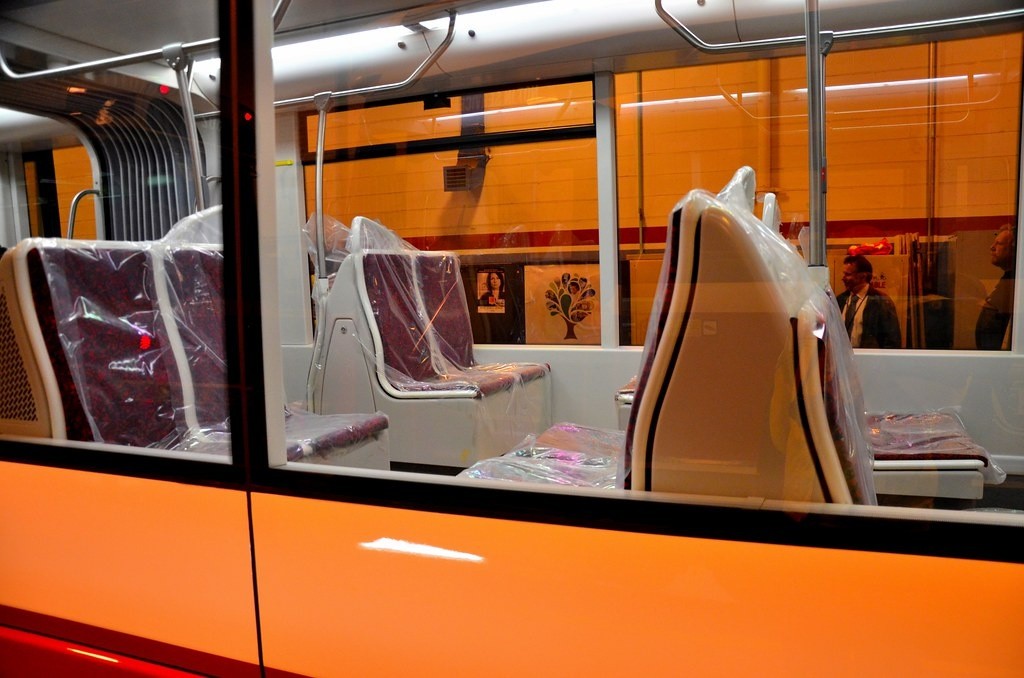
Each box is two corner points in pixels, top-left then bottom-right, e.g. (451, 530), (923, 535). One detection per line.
(478, 272), (505, 306)
(836, 254), (901, 349)
(975, 224), (1017, 350)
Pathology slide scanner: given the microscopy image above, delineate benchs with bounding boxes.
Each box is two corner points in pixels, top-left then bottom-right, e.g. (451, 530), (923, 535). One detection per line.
(1, 166), (1024, 515)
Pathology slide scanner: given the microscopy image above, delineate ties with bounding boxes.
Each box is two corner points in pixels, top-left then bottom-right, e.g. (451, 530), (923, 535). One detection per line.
(845, 295), (859, 343)
